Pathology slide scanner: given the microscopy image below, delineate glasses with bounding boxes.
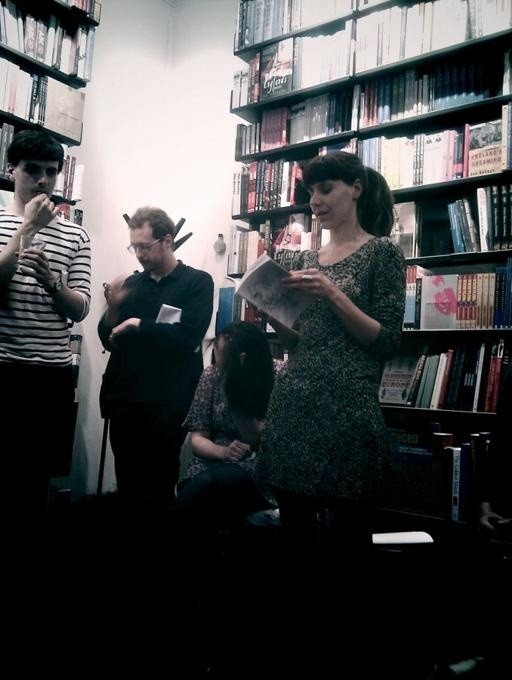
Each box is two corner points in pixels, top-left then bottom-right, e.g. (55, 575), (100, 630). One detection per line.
(127, 235), (165, 255)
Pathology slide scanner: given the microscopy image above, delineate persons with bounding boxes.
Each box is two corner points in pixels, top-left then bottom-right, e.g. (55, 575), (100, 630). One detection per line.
(97, 207), (214, 501)
(176, 322), (280, 514)
(246, 150), (406, 546)
(0, 132), (90, 479)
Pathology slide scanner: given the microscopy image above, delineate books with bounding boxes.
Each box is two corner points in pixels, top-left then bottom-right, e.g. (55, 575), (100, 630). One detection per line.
(0, 0), (101, 388)
(357, 53), (512, 190)
(227, 84), (360, 375)
(402, 258), (512, 330)
(232, 0), (511, 108)
(379, 337), (511, 520)
(390, 185), (512, 259)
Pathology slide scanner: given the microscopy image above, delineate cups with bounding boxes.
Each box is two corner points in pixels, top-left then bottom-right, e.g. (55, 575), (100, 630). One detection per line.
(18, 235), (47, 273)
